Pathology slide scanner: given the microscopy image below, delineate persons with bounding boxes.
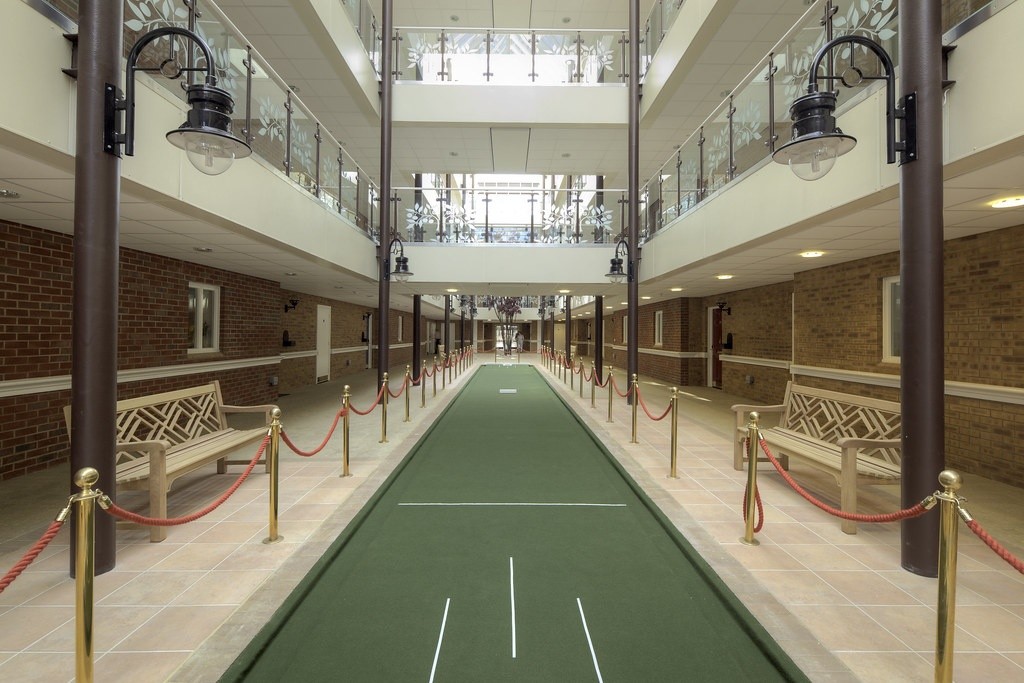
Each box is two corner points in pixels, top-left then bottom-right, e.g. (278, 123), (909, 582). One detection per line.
(433, 329), (441, 354)
(516, 331), (524, 353)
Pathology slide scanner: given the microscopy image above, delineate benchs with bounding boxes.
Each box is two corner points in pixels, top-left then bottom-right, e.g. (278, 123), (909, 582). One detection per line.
(562, 344), (577, 368)
(63, 377), (280, 543)
(438, 345), (445, 360)
(732, 380), (899, 534)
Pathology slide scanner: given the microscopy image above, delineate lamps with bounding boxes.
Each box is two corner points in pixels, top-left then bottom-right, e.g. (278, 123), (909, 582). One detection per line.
(387, 237), (412, 284)
(769, 35), (917, 179)
(106, 25), (252, 175)
(716, 297), (731, 315)
(363, 310), (373, 320)
(606, 238), (628, 286)
(284, 295), (300, 314)
